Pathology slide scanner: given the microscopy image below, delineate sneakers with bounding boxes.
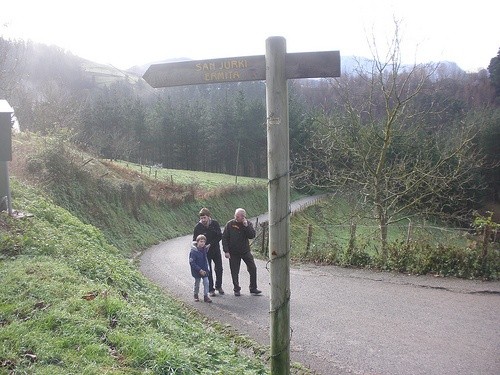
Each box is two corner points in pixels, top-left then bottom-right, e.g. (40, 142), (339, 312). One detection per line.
(204, 296), (212, 302)
(250, 288), (262, 293)
(194, 294), (199, 302)
(234, 291), (240, 296)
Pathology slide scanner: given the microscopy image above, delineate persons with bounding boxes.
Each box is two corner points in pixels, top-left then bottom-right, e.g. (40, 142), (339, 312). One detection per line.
(193, 208), (224, 296)
(222, 208), (262, 295)
(189, 235), (212, 303)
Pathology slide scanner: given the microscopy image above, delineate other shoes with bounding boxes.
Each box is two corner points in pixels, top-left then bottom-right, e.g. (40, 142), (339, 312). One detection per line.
(216, 287), (224, 294)
(210, 290), (215, 296)
